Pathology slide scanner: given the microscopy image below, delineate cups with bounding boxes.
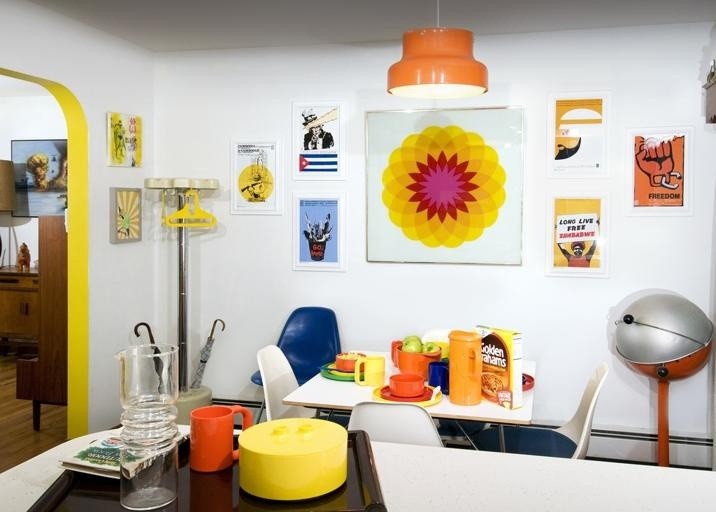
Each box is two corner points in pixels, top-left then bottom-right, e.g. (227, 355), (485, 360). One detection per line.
(189, 405), (253, 472)
(428, 362), (449, 395)
(355, 355), (385, 387)
(448, 330), (482, 406)
(119, 344), (180, 510)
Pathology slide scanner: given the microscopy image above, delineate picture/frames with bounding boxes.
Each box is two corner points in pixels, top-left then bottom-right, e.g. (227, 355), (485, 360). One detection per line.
(546, 88), (612, 182)
(107, 111), (143, 167)
(12, 141), (67, 217)
(548, 191), (610, 279)
(291, 101), (346, 186)
(231, 137), (279, 215)
(292, 194), (346, 271)
(365, 106), (524, 266)
(109, 187), (142, 243)
(627, 126), (693, 218)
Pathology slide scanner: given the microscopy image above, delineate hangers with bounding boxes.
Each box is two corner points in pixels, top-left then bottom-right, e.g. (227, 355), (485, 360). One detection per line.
(166, 189), (216, 228)
(158, 189), (169, 227)
(145, 176), (216, 425)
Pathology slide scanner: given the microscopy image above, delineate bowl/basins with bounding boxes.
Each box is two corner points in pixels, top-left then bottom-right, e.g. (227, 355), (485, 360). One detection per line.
(396, 345), (442, 381)
(389, 374), (425, 397)
(334, 353), (367, 371)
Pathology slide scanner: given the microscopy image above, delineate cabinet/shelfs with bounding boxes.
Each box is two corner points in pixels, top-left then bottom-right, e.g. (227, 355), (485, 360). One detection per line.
(16, 216), (67, 432)
(0, 267), (40, 356)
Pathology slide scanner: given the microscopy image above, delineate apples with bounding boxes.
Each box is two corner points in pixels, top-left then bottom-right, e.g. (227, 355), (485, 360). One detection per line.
(422, 342), (440, 353)
(401, 336), (422, 352)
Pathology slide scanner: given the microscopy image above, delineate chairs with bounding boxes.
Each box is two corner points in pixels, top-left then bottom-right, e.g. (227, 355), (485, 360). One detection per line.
(257, 345), (350, 428)
(473, 363), (610, 459)
(348, 402), (444, 447)
(251, 307), (340, 424)
(437, 419), (491, 447)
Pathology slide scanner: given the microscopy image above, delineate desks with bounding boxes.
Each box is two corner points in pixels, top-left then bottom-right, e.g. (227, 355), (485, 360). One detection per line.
(0, 426), (716, 512)
(281, 351), (537, 452)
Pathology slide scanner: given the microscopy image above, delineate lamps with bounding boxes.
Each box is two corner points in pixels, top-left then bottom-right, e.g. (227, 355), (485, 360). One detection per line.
(385, 0), (488, 101)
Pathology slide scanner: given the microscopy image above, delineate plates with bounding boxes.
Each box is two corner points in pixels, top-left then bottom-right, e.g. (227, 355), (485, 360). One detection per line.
(380, 385), (433, 402)
(320, 362), (363, 382)
(373, 387), (442, 408)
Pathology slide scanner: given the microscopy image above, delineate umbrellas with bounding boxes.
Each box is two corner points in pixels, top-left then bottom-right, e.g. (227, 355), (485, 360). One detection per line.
(190, 318), (226, 389)
(133, 322), (171, 394)
